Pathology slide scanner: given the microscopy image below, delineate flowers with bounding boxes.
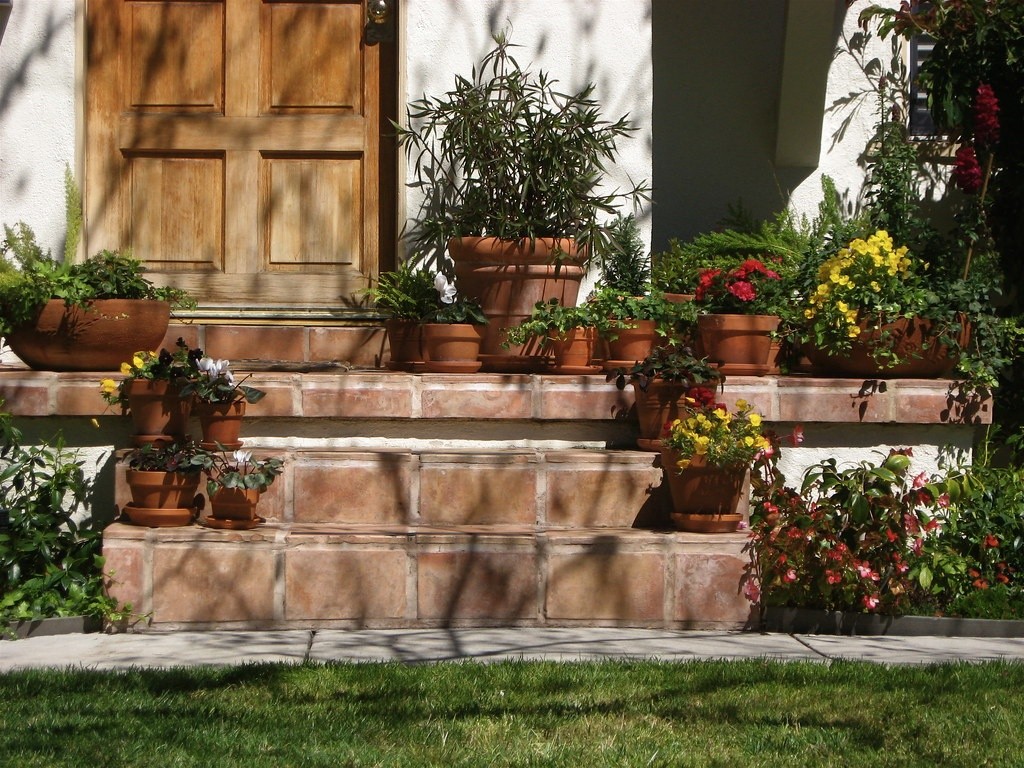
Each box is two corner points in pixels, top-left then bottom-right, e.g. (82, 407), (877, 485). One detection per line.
(91, 335), (267, 429)
(695, 258), (781, 317)
(419, 271), (492, 326)
(788, 228), (969, 370)
(658, 388), (772, 470)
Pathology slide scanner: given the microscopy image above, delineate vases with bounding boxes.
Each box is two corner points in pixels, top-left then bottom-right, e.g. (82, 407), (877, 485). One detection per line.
(794, 310), (972, 378)
(697, 313), (784, 373)
(421, 323), (486, 373)
(659, 447), (749, 533)
(198, 401), (247, 452)
(125, 379), (193, 450)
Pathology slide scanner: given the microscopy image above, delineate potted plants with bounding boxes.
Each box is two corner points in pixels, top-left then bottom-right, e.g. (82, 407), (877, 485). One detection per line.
(607, 338), (727, 450)
(351, 248), (445, 373)
(0, 162), (198, 370)
(190, 438), (286, 530)
(121, 436), (205, 527)
(380, 16), (722, 376)
